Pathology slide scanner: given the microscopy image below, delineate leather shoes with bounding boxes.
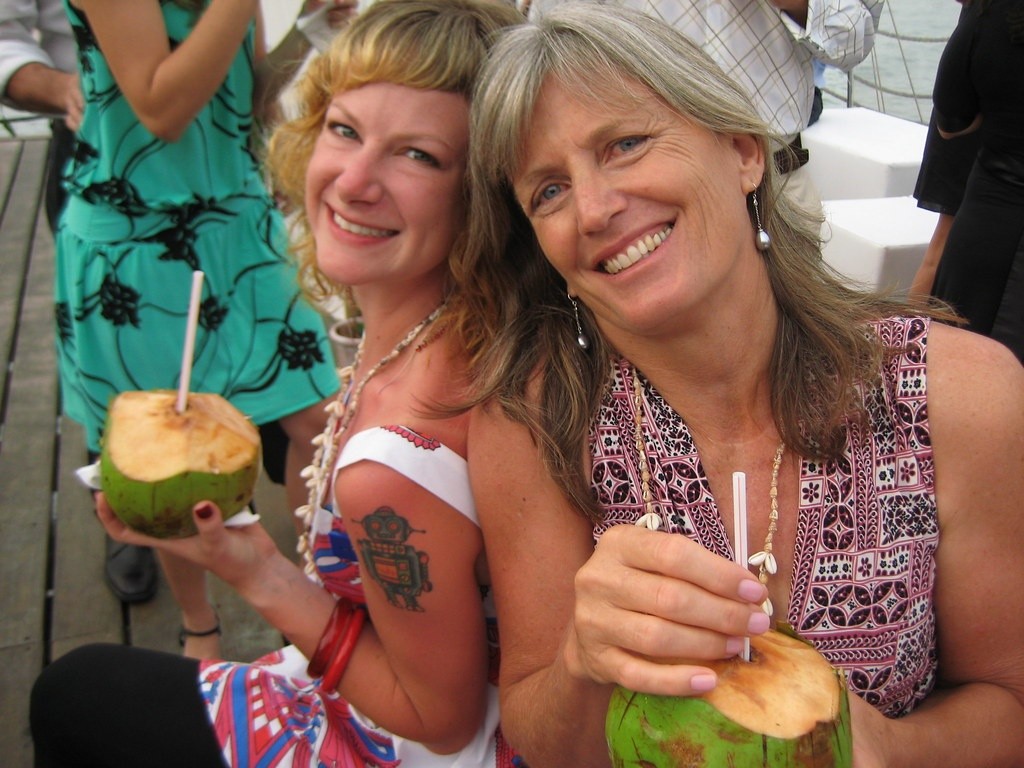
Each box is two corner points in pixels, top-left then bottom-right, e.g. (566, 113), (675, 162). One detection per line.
(105, 532), (159, 600)
(260, 421), (290, 484)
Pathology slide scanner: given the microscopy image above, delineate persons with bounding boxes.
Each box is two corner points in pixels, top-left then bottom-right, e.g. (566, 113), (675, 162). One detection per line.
(409, 4), (1024, 767)
(516, 0), (884, 245)
(928, 0), (1024, 364)
(49, 1), (356, 661)
(28, 0), (541, 768)
(1, 0), (285, 608)
(902, 106), (981, 302)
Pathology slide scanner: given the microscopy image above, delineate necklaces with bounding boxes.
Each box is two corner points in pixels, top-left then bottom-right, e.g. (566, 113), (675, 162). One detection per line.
(292, 295), (460, 594)
(632, 367), (786, 617)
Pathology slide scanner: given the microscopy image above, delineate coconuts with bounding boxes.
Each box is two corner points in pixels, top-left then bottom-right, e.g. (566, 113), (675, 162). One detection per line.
(606, 629), (853, 768)
(100, 391), (260, 539)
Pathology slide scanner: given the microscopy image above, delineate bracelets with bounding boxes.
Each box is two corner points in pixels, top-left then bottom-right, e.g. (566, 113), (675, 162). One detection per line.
(320, 608), (366, 692)
(306, 597), (352, 680)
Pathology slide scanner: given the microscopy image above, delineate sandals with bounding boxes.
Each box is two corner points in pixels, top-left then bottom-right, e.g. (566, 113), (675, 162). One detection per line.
(180, 607), (222, 647)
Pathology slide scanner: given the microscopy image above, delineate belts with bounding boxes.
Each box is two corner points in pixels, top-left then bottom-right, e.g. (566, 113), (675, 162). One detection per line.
(774, 132), (809, 174)
